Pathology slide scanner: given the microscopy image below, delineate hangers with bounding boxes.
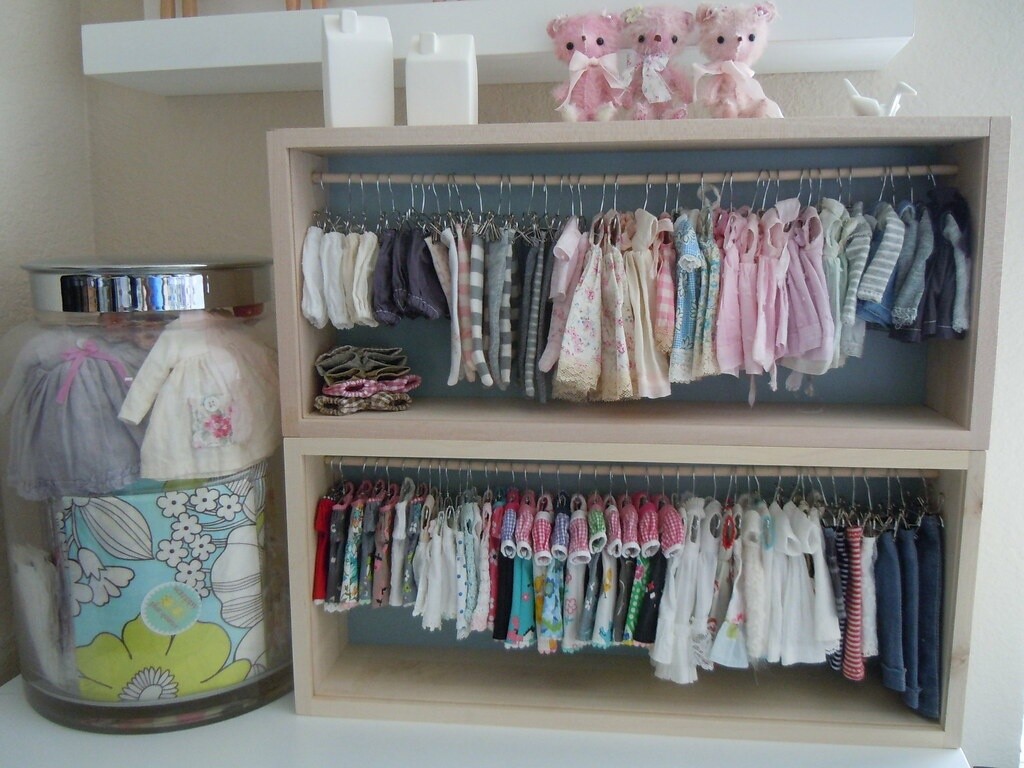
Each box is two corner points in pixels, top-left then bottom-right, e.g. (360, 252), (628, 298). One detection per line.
(328, 455), (947, 541)
(311, 162), (941, 250)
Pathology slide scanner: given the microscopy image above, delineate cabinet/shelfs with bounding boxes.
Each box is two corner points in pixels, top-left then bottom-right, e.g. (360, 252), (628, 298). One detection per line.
(267, 113), (1013, 750)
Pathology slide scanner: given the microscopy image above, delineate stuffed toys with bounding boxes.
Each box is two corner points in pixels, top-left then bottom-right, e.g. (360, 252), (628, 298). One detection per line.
(619, 8), (694, 120)
(693, 0), (783, 118)
(548, 14), (620, 122)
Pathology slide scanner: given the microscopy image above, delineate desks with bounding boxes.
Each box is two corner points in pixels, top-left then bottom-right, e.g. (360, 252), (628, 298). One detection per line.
(0, 675), (976, 768)
